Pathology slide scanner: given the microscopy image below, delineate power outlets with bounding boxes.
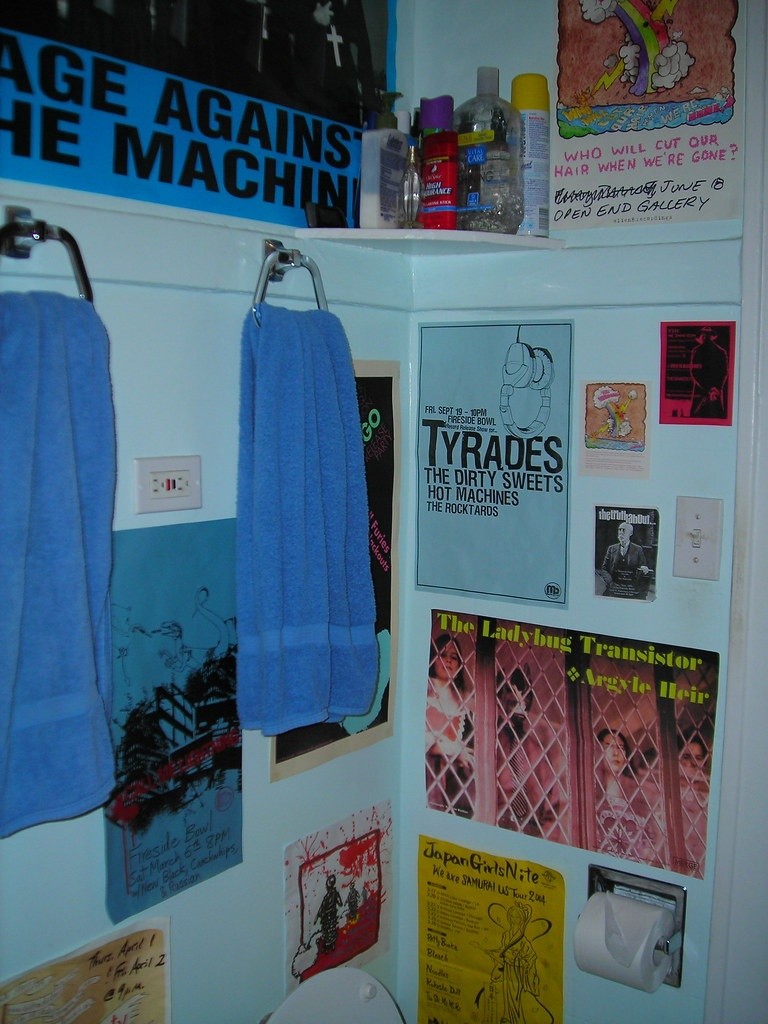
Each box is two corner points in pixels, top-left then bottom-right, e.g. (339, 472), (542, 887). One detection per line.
(132, 454), (203, 515)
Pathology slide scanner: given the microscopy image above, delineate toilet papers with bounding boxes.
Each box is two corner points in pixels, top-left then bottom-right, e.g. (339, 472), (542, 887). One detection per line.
(574, 891), (677, 993)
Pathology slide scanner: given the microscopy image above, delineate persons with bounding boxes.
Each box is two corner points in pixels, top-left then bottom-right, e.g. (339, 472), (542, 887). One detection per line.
(424, 632), (710, 880)
(0, 0), (380, 131)
(601, 521), (650, 600)
(689, 326), (728, 418)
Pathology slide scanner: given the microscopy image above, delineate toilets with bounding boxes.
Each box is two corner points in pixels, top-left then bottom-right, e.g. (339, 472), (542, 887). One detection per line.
(258, 962), (401, 1024)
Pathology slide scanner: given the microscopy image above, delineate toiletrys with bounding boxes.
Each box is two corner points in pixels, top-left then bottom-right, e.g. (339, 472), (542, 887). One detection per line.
(362, 67), (549, 237)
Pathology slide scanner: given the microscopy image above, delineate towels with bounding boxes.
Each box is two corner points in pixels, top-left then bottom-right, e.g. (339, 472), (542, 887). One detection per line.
(233, 302), (381, 736)
(0, 288), (119, 840)
